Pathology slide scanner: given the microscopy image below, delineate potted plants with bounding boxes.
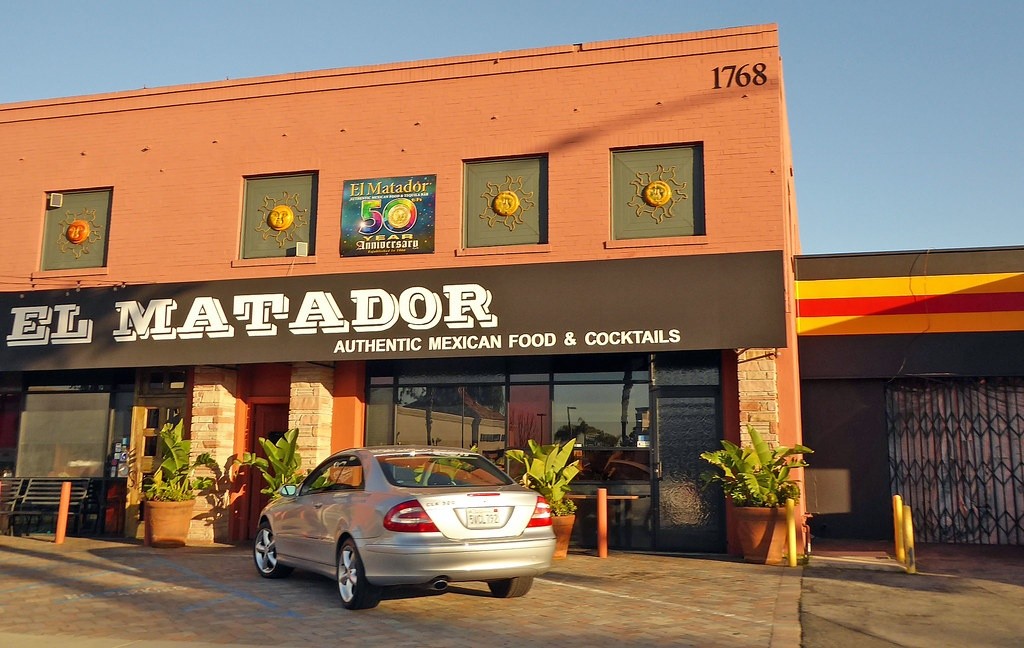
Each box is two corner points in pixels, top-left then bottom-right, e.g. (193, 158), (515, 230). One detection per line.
(510, 438), (584, 560)
(140, 419), (221, 546)
(704, 425), (815, 563)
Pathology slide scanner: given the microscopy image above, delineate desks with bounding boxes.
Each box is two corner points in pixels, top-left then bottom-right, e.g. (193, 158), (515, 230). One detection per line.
(564, 488), (648, 558)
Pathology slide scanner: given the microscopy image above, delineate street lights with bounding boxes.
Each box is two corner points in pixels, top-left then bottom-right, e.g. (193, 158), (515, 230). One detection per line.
(537, 413), (546, 446)
(567, 406), (576, 442)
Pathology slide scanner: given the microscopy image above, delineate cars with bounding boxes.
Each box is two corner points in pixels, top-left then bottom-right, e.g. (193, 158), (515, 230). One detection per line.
(253, 445), (558, 611)
(557, 460), (697, 533)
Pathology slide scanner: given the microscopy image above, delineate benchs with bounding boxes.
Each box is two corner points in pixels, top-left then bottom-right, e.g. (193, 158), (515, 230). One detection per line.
(0, 476), (93, 536)
